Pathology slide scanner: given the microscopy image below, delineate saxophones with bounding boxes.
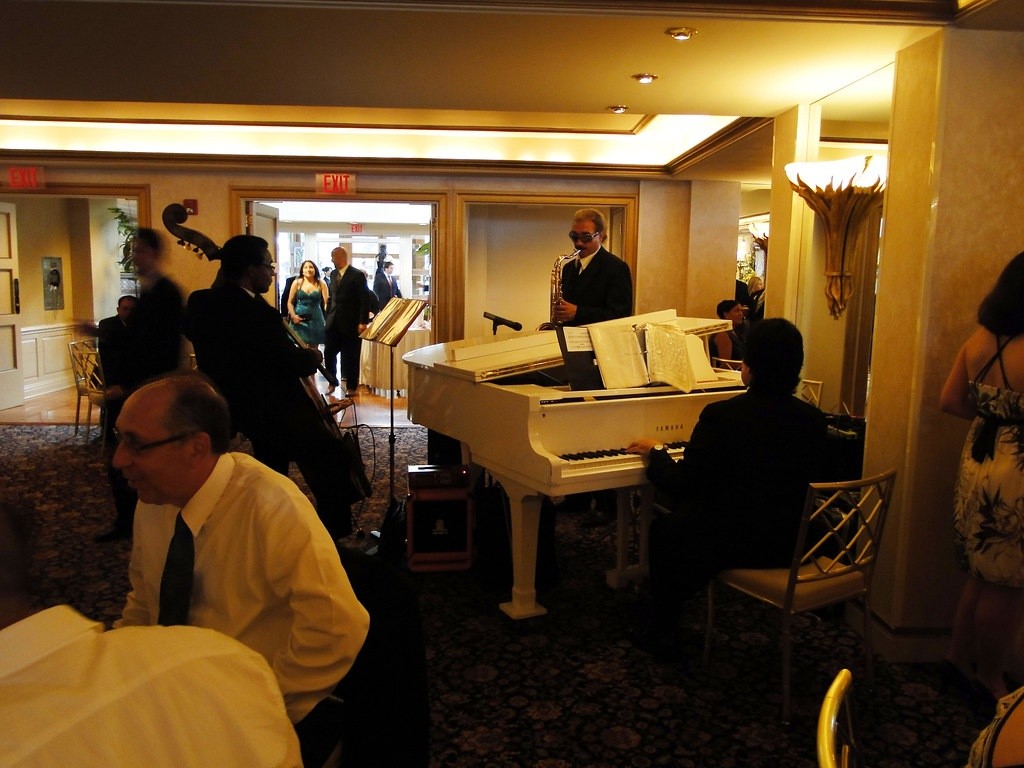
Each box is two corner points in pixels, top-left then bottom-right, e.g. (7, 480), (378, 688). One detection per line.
(535, 249), (581, 331)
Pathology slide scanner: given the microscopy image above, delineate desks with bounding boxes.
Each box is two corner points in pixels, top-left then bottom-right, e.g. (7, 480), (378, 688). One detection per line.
(360, 324), (431, 396)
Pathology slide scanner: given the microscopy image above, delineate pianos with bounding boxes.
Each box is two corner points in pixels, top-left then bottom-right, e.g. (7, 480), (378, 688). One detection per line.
(401, 308), (750, 621)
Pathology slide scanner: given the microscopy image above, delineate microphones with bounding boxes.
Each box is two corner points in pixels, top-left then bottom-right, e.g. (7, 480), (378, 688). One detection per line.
(483, 312), (523, 331)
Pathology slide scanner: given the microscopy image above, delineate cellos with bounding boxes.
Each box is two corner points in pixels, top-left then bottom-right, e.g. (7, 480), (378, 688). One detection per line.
(162, 203), (373, 540)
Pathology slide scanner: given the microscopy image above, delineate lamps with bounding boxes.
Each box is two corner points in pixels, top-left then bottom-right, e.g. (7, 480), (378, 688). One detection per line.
(784, 148), (889, 318)
(747, 220), (769, 255)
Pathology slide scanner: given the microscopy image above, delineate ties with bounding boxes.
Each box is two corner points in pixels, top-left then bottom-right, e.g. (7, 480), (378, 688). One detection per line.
(575, 262), (583, 279)
(157, 510), (194, 626)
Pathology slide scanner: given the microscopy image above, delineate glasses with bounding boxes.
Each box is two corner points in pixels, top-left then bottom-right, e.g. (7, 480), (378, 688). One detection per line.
(245, 260), (276, 272)
(112, 422), (208, 456)
(568, 230), (600, 242)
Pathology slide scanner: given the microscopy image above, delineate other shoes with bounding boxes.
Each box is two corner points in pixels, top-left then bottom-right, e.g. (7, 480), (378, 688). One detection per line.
(581, 509), (612, 526)
(93, 524), (133, 540)
(556, 497), (580, 512)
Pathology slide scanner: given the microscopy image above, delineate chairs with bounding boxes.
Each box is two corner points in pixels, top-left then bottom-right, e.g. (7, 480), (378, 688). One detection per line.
(66, 339), (99, 434)
(696, 469), (898, 731)
(77, 347), (121, 454)
(300, 562), (424, 768)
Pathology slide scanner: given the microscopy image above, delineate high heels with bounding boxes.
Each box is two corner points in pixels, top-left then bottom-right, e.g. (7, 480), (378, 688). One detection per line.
(967, 678), (1000, 716)
(941, 661), (973, 690)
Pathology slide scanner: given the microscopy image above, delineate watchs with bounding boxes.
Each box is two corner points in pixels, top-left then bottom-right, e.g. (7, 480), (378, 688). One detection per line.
(648, 442), (664, 458)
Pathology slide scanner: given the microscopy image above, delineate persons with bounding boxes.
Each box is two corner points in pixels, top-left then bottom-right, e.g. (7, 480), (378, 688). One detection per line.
(938, 252), (1024, 701)
(0, 472), (300, 768)
(98, 228), (402, 541)
(707, 299), (747, 370)
(548, 209), (633, 528)
(624, 318), (828, 663)
(108, 376), (372, 728)
